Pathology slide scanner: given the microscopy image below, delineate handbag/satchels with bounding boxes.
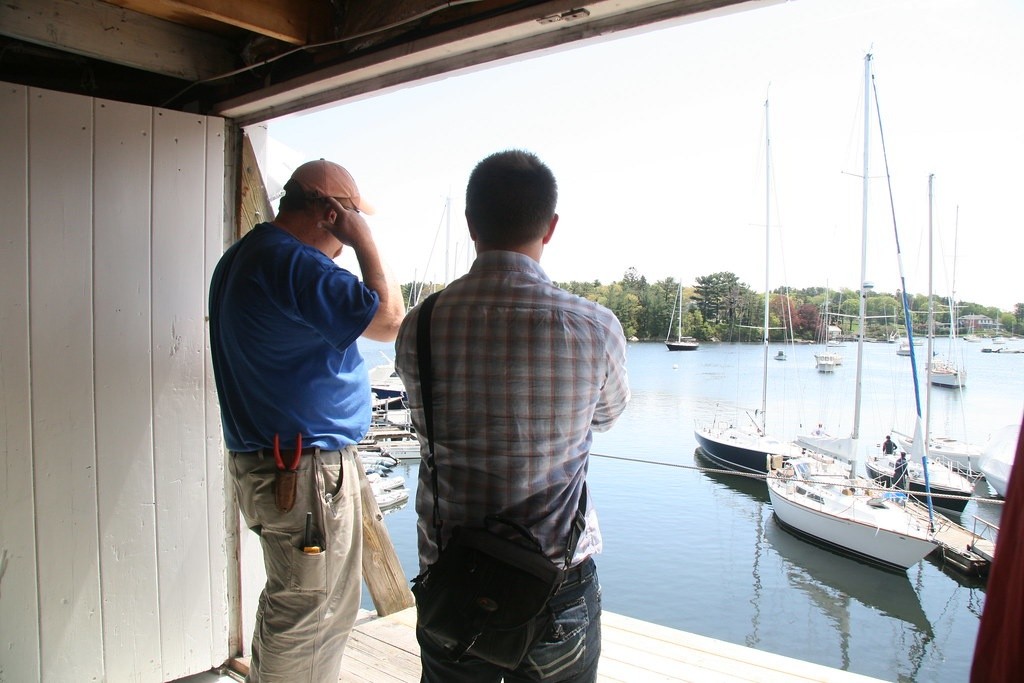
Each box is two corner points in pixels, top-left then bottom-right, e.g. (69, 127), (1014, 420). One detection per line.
(407, 520), (566, 671)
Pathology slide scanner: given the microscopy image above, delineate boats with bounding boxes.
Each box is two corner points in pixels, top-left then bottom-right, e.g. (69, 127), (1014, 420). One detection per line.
(359, 445), (421, 510)
(774, 350), (787, 361)
(977, 424), (1021, 499)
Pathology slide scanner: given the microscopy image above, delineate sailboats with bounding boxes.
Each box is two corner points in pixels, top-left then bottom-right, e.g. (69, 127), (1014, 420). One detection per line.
(366, 198), (451, 410)
(814, 277), (846, 374)
(867, 172), (985, 517)
(764, 54), (954, 574)
(693, 99), (804, 485)
(890, 203), (988, 477)
(664, 277), (699, 352)
(896, 286), (1019, 388)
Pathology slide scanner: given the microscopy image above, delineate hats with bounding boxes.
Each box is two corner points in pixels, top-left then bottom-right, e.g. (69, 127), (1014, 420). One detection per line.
(286, 158), (374, 215)
(901, 452), (906, 456)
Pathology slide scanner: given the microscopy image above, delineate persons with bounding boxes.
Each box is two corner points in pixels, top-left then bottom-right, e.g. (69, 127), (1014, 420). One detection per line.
(207, 157), (405, 682)
(893, 452), (909, 491)
(883, 435), (898, 455)
(394, 152), (631, 683)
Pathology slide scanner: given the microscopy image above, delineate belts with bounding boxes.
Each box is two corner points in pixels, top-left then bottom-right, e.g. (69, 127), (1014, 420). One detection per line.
(560, 558), (596, 585)
(246, 445), (315, 458)
